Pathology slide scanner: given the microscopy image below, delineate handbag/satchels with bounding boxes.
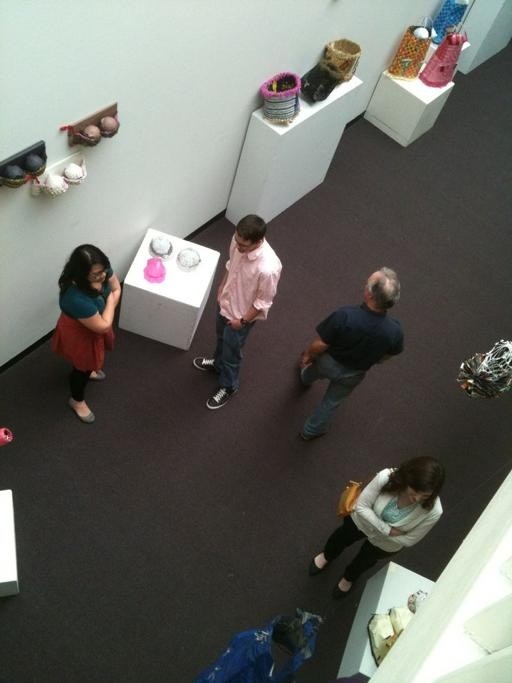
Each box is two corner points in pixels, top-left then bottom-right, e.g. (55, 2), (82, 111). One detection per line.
(335, 480), (363, 519)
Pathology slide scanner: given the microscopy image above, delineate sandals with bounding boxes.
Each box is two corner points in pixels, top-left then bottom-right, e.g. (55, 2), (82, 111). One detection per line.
(88, 369), (106, 381)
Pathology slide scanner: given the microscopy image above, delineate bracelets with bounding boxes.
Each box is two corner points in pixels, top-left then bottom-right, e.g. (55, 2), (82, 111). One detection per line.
(240, 318), (249, 328)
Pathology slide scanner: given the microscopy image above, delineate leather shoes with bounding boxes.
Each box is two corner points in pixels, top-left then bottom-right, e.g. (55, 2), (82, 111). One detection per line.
(337, 575), (353, 599)
(68, 397), (95, 423)
(309, 552), (329, 576)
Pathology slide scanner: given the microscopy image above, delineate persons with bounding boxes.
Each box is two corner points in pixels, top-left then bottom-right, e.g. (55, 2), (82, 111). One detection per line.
(192, 211), (284, 410)
(51, 242), (123, 426)
(294, 263), (405, 442)
(306, 455), (444, 599)
(195, 609), (321, 682)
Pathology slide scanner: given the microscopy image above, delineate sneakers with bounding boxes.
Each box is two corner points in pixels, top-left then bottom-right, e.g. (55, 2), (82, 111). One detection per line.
(206, 385), (237, 410)
(298, 350), (315, 388)
(192, 357), (216, 370)
(299, 421), (332, 441)
